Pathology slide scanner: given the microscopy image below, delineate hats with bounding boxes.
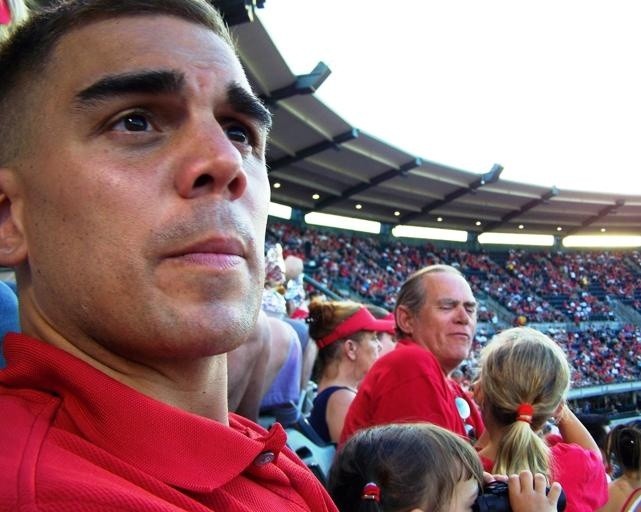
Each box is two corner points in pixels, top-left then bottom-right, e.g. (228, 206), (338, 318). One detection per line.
(317, 307), (397, 349)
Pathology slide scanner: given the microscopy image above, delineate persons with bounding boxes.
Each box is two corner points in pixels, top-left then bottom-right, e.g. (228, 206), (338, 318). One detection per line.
(249, 218), (641, 512)
(0, 0), (340, 512)
